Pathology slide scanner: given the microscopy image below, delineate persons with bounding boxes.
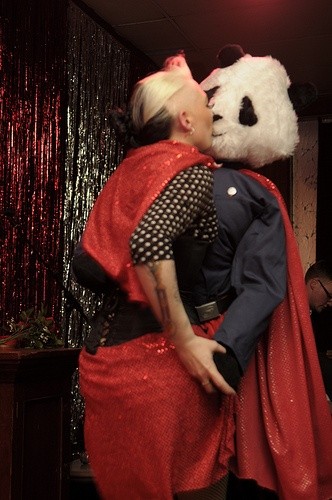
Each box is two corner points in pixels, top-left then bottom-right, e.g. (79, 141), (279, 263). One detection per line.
(71, 70), (236, 500)
(298, 263), (332, 319)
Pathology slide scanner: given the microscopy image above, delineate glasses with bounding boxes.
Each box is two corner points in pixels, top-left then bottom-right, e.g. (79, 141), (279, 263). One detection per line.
(317, 279), (332, 301)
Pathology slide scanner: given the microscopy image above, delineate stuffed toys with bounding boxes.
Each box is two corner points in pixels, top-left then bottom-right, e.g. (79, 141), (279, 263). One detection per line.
(201, 45), (331, 500)
